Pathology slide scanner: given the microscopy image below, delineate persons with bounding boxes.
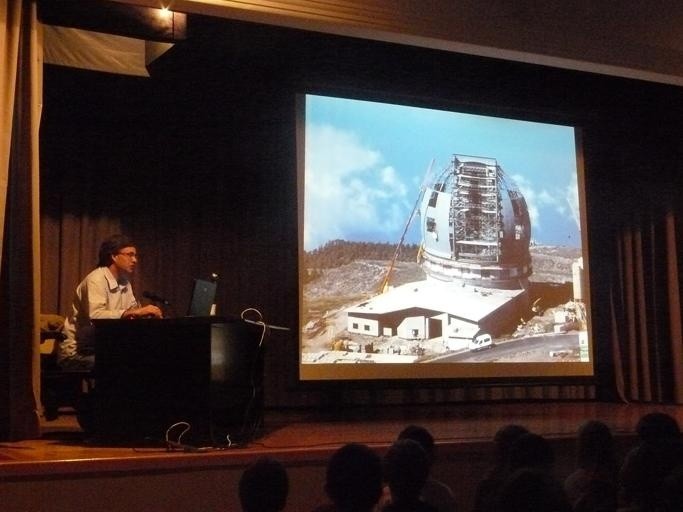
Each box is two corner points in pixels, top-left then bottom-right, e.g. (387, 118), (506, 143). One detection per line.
(323, 412), (683, 511)
(52, 233), (163, 431)
(239, 456), (289, 512)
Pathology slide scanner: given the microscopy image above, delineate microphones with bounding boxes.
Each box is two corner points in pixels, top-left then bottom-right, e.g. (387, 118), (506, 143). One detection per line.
(142, 289), (170, 305)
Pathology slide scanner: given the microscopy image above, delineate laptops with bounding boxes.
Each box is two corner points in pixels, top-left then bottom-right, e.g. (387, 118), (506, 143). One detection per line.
(180, 277), (217, 316)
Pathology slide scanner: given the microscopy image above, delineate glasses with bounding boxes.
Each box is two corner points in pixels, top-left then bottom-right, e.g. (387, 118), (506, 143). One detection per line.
(119, 251), (138, 259)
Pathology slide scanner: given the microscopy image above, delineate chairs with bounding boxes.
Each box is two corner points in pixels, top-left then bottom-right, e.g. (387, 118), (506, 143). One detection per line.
(40, 315), (93, 422)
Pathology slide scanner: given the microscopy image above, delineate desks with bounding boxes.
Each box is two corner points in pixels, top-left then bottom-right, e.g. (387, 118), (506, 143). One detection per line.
(91, 317), (265, 445)
